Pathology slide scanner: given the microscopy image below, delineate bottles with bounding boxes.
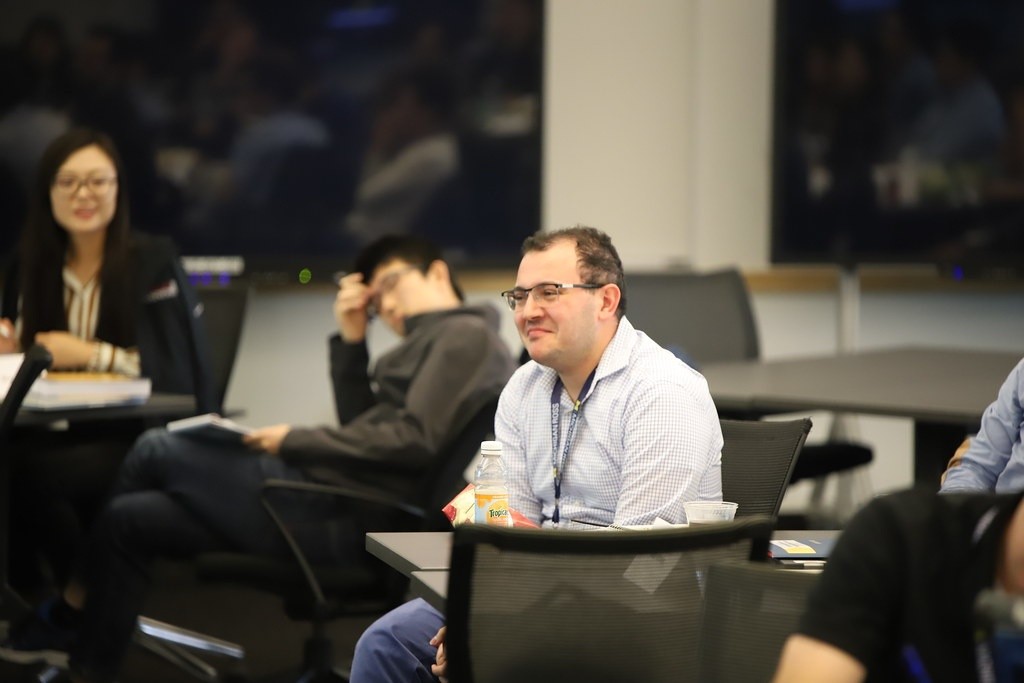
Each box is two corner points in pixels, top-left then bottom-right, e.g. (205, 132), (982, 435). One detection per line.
(474, 441), (510, 527)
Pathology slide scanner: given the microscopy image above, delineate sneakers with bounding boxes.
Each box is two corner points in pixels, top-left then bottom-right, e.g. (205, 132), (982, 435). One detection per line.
(0, 596), (78, 672)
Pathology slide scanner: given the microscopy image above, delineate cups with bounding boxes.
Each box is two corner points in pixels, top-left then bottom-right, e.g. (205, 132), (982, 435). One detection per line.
(683, 501), (739, 528)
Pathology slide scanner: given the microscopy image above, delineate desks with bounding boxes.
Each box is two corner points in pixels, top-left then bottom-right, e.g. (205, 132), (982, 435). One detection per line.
(683, 339), (1024, 486)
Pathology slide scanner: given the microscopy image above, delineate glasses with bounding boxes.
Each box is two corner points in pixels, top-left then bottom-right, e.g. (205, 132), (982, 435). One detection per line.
(53, 169), (116, 200)
(501, 279), (609, 309)
(365, 261), (422, 320)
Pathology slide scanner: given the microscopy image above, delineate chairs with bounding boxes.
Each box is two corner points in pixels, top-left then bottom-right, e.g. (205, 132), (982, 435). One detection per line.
(111, 61), (977, 270)
(616, 265), (872, 539)
(193, 477), (431, 682)
(418, 512), (784, 681)
(167, 252), (248, 425)
(703, 416), (816, 559)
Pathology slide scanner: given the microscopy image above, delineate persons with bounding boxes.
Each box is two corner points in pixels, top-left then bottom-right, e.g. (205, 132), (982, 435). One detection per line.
(1, 130), (212, 608)
(768, 490), (1023, 682)
(350, 229), (725, 682)
(3, 231), (523, 682)
(937, 357), (1023, 494)
